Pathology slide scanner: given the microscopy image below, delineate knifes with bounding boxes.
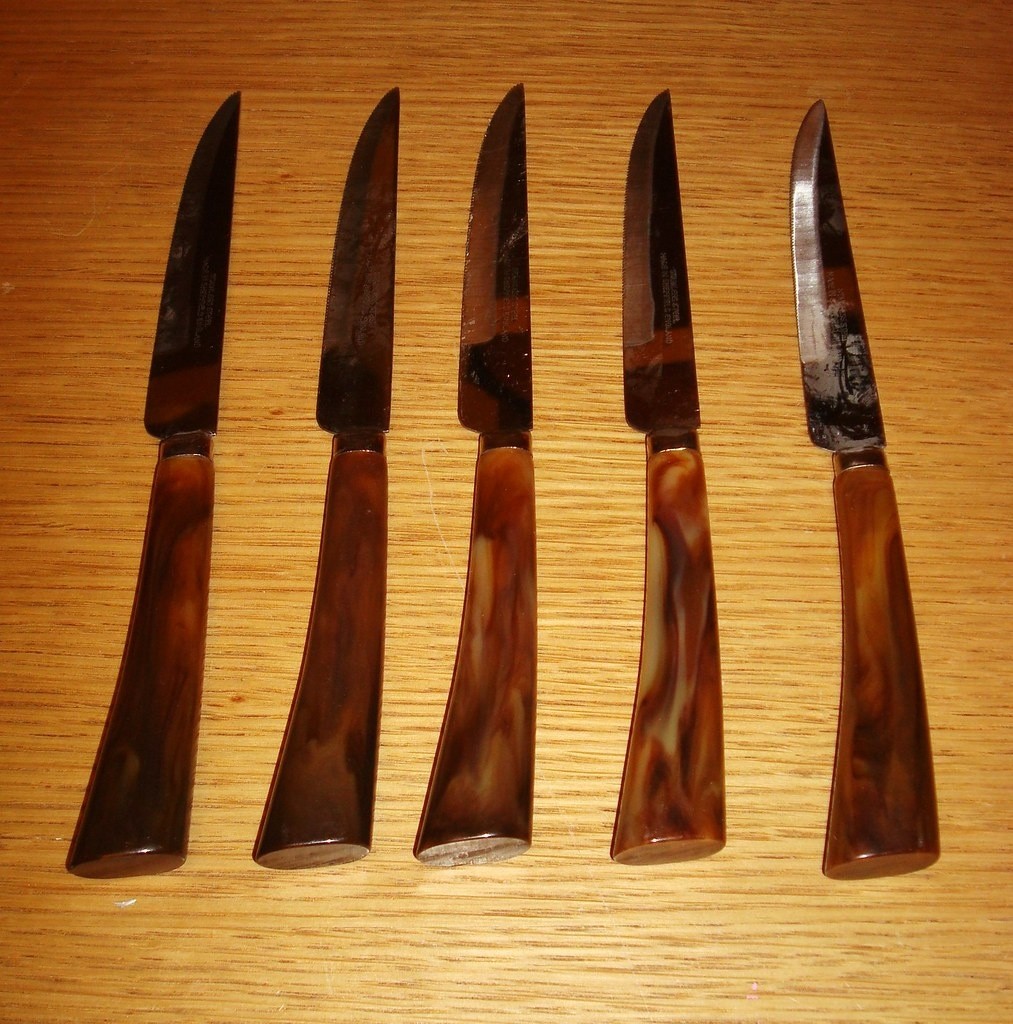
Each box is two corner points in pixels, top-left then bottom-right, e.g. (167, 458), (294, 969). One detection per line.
(413, 82), (536, 869)
(609, 88), (725, 865)
(252, 88), (400, 870)
(66, 90), (241, 879)
(790, 100), (942, 879)
